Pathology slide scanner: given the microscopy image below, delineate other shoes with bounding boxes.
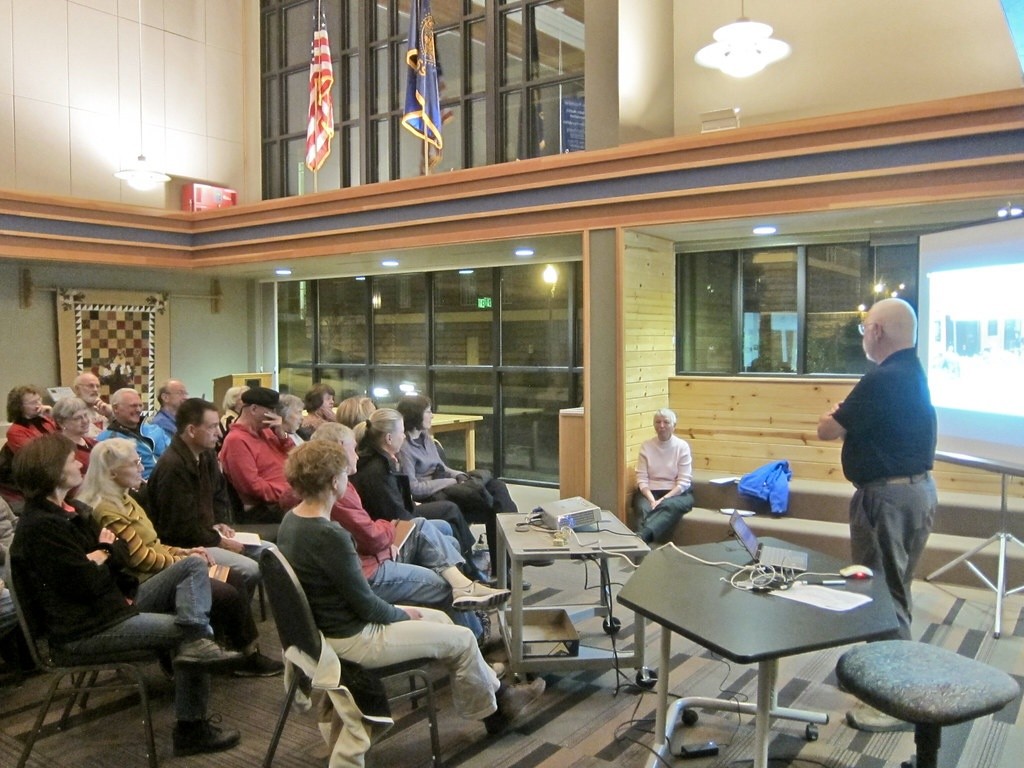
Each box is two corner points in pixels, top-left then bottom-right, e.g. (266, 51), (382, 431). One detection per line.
(523, 558), (554, 568)
(236, 652), (284, 677)
(505, 568), (530, 590)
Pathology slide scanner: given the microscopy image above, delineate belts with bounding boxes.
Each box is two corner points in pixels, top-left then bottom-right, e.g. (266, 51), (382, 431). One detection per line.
(852, 471), (933, 488)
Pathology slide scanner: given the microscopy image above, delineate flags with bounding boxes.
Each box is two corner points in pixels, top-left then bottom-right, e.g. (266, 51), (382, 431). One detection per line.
(306, 0), (334, 171)
(402, 0), (442, 150)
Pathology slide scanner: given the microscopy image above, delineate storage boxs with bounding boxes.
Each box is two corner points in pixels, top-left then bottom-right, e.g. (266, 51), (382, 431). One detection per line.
(504, 609), (580, 658)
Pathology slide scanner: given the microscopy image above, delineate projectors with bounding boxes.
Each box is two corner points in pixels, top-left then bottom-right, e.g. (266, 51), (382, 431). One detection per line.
(539, 496), (602, 531)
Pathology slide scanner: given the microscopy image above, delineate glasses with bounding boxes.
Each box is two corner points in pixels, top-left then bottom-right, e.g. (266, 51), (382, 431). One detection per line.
(858, 323), (872, 335)
(120, 457), (142, 468)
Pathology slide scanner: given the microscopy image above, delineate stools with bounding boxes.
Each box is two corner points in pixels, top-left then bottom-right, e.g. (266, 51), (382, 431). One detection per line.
(835, 640), (1021, 768)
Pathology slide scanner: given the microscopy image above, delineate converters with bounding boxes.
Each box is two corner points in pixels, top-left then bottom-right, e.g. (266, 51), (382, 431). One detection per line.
(680, 740), (719, 758)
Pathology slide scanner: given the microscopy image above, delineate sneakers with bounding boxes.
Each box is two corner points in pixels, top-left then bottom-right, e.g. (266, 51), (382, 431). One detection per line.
(500, 678), (546, 722)
(483, 711), (508, 734)
(172, 723), (241, 756)
(174, 638), (241, 668)
(451, 579), (511, 611)
(846, 705), (918, 731)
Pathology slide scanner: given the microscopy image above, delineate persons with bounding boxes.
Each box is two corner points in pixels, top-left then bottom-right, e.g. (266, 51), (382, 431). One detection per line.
(819, 299), (937, 732)
(5, 430), (243, 754)
(216, 384), (376, 519)
(310, 421), (513, 679)
(97, 388), (170, 489)
(635, 410), (694, 543)
(74, 373), (115, 439)
(353, 397), (517, 585)
(0, 497), (39, 674)
(275, 442), (545, 736)
(153, 379), (188, 438)
(79, 437), (284, 676)
(143, 398), (278, 602)
(55, 397), (102, 478)
(6, 386), (56, 451)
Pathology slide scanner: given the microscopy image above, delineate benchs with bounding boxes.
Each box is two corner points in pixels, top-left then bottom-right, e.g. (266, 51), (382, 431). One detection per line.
(669, 472), (1024, 600)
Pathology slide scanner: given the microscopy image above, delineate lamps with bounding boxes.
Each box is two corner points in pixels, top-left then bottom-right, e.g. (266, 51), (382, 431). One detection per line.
(111, 0), (172, 192)
(695, 0), (791, 75)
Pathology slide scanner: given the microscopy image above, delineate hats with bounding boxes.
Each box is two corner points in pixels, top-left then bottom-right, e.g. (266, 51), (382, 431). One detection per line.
(242, 387), (282, 410)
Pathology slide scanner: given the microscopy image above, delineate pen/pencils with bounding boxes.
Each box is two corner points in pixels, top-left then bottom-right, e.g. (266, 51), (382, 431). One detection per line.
(802, 580), (845, 585)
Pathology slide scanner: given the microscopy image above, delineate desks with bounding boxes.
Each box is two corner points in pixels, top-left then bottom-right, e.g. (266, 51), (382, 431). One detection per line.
(303, 406), (485, 473)
(494, 510), (658, 690)
(617, 536), (899, 768)
(381, 403), (544, 473)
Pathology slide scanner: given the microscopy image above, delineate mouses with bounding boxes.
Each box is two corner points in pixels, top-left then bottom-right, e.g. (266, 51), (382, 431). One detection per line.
(840, 565), (873, 579)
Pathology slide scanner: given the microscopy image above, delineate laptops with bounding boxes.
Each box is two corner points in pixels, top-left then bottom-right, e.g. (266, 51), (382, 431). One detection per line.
(730, 509), (808, 573)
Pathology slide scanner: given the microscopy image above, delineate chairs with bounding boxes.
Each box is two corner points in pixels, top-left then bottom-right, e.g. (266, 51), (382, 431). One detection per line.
(259, 547), (442, 768)
(7, 544), (159, 768)
(220, 468), (284, 622)
